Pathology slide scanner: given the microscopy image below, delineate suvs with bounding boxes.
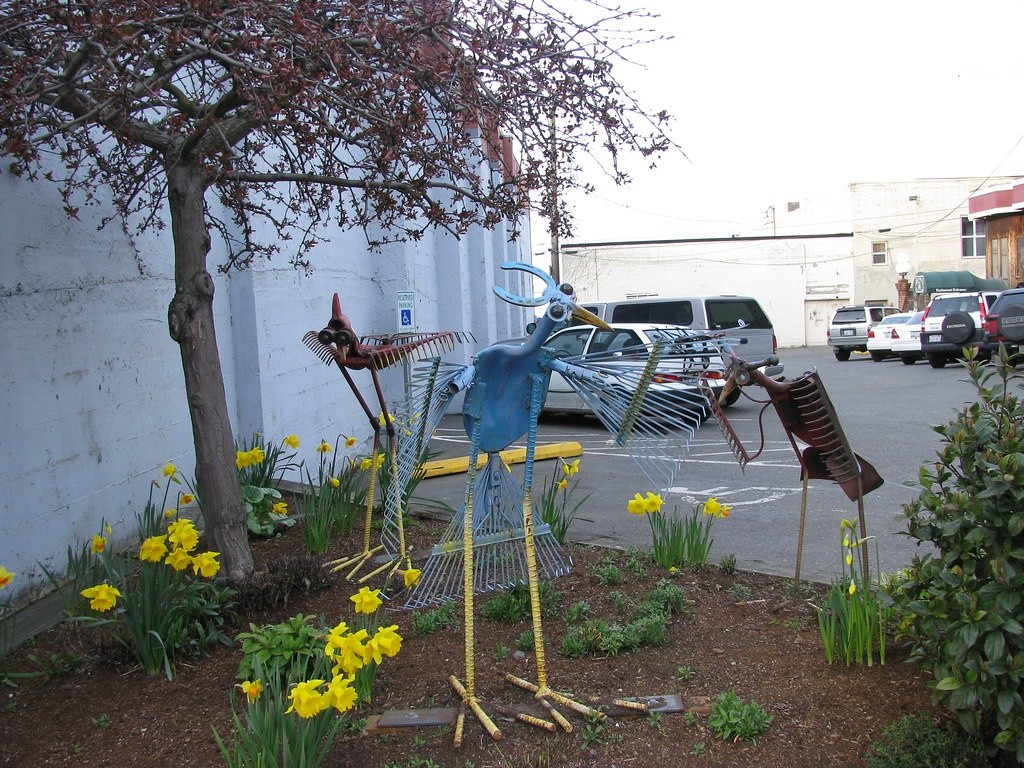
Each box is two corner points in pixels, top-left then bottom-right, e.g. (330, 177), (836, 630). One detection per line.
(526, 291), (784, 407)
(919, 290), (995, 369)
(980, 284), (1024, 370)
(826, 303), (904, 361)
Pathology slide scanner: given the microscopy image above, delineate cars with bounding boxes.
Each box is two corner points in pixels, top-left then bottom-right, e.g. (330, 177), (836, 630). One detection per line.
(889, 310), (928, 366)
(866, 312), (914, 362)
(540, 321), (729, 433)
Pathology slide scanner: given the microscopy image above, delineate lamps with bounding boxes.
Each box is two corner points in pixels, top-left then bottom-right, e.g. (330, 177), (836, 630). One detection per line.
(895, 252), (911, 278)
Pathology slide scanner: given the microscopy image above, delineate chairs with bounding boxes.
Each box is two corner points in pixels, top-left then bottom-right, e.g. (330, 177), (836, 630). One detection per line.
(622, 338), (638, 355)
(580, 342), (609, 362)
